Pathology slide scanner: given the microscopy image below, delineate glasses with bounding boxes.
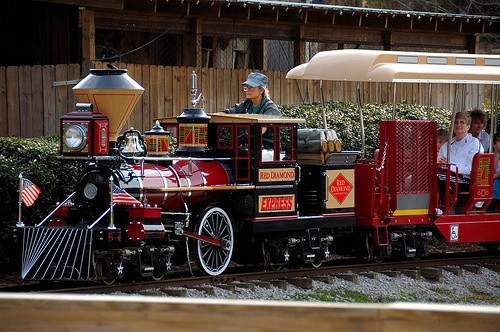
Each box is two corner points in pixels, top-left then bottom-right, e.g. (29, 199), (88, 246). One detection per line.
(455, 121), (468, 124)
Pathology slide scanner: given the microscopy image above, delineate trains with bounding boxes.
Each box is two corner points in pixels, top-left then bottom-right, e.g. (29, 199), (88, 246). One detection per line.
(10, 47), (500, 288)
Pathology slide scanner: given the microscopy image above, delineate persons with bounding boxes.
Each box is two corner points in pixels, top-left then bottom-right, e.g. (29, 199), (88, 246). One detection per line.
(436, 111), (485, 203)
(467, 108), (492, 161)
(213, 71), (281, 161)
(486, 131), (500, 201)
(434, 128), (450, 159)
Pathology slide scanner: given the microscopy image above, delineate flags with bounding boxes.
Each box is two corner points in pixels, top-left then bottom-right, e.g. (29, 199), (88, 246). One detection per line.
(21, 178), (42, 206)
(112, 183), (141, 205)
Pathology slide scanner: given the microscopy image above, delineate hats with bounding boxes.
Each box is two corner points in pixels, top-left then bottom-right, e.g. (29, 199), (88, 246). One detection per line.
(241, 73), (268, 89)
